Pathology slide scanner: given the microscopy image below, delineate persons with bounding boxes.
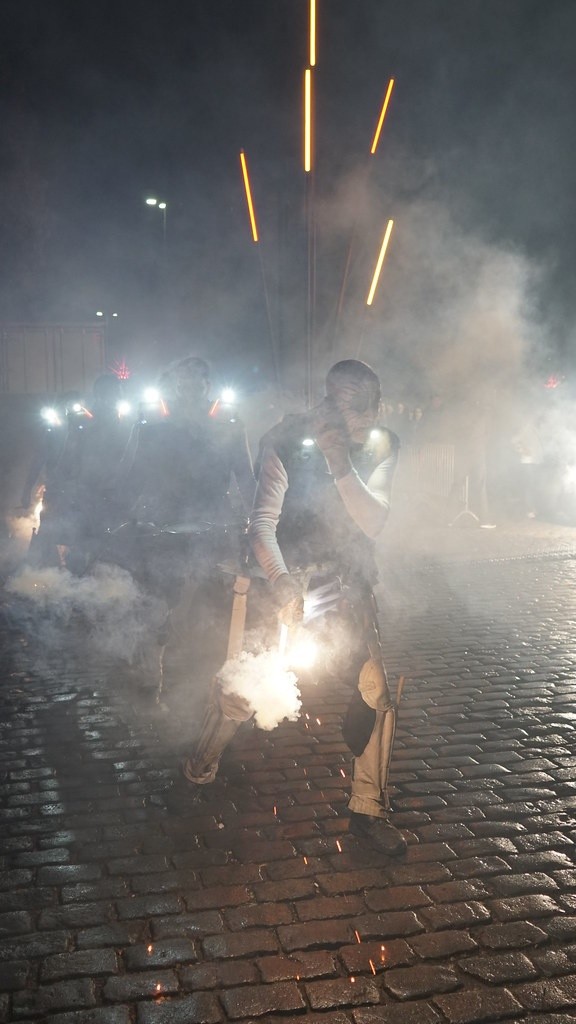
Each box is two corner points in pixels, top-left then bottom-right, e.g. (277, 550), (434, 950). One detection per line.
(11, 358), (256, 713)
(184, 360), (407, 855)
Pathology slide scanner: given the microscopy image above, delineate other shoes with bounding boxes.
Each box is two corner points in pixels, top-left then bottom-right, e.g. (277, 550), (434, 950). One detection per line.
(349, 815), (409, 857)
(166, 777), (203, 813)
(138, 688), (161, 708)
